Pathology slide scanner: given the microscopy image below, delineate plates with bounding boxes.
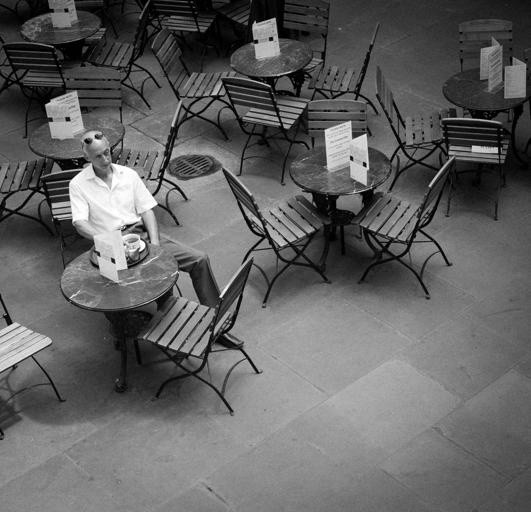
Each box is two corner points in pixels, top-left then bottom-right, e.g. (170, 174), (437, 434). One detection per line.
(139, 240), (145, 253)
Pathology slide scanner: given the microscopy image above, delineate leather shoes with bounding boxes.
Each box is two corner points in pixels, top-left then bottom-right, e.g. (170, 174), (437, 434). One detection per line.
(215, 332), (244, 348)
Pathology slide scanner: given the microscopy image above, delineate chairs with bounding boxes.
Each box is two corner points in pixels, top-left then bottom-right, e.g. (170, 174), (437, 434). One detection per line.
(0, 293), (66, 440)
(137, 256), (262, 416)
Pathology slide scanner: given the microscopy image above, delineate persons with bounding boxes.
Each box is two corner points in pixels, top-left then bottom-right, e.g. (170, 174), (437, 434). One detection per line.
(68, 129), (245, 349)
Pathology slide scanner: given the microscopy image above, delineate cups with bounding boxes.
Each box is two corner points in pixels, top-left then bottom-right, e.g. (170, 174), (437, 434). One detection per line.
(123, 233), (141, 250)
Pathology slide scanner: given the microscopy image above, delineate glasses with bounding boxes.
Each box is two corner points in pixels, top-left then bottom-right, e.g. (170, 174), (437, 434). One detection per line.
(82, 133), (103, 150)
